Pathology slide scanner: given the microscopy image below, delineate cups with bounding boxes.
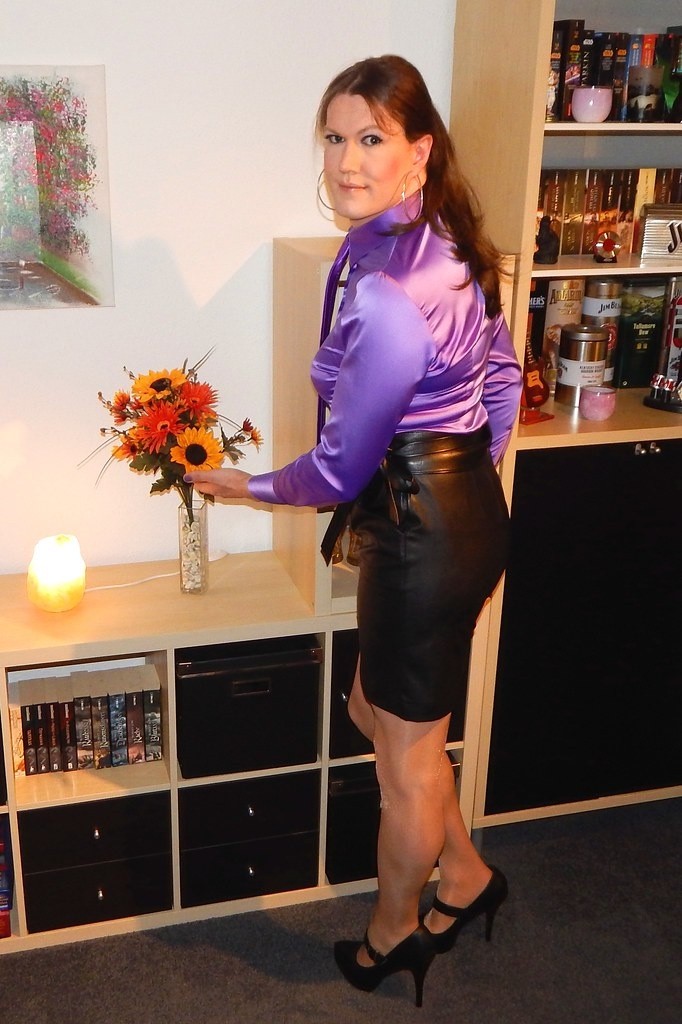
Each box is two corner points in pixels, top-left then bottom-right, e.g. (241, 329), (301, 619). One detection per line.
(571, 84), (615, 124)
(578, 386), (617, 421)
(626, 64), (664, 123)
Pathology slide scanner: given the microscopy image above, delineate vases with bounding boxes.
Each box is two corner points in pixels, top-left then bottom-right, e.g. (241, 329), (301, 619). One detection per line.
(179, 499), (210, 596)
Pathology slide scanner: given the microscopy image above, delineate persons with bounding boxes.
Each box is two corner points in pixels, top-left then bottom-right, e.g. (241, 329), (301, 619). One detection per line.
(179, 54), (525, 1007)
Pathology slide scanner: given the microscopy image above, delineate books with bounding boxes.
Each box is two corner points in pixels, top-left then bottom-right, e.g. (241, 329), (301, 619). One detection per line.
(535, 165), (682, 260)
(543, 16), (682, 124)
(7, 663), (166, 778)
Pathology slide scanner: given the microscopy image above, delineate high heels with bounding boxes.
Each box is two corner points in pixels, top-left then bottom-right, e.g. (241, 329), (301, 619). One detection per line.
(419, 863), (508, 954)
(334, 924), (437, 1007)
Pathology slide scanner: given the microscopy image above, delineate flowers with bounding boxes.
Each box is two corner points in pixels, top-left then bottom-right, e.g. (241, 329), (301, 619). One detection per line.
(0, 75), (102, 263)
(77, 344), (264, 532)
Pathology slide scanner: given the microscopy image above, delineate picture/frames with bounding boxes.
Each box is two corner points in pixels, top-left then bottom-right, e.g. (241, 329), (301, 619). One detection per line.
(0, 63), (115, 309)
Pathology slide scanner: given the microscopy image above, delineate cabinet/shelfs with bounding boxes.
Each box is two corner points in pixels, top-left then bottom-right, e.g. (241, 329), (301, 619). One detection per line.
(0, 0), (682, 961)
